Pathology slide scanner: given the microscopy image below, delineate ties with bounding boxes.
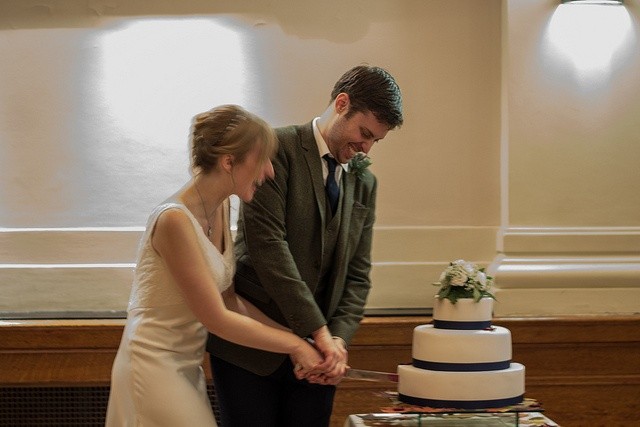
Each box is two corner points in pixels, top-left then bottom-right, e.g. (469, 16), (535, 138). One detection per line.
(322, 153), (340, 220)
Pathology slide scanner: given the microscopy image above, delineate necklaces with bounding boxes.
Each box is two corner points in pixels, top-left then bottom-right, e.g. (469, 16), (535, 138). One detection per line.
(193, 176), (218, 237)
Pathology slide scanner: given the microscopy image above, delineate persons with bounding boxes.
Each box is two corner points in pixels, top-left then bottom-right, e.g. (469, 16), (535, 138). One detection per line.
(104, 105), (348, 426)
(205, 65), (403, 424)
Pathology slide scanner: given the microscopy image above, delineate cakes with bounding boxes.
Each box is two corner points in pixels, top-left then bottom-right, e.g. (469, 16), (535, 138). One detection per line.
(396, 295), (527, 408)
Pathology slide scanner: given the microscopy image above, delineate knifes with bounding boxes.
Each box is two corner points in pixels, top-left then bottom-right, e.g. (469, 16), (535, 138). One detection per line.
(340, 365), (401, 385)
